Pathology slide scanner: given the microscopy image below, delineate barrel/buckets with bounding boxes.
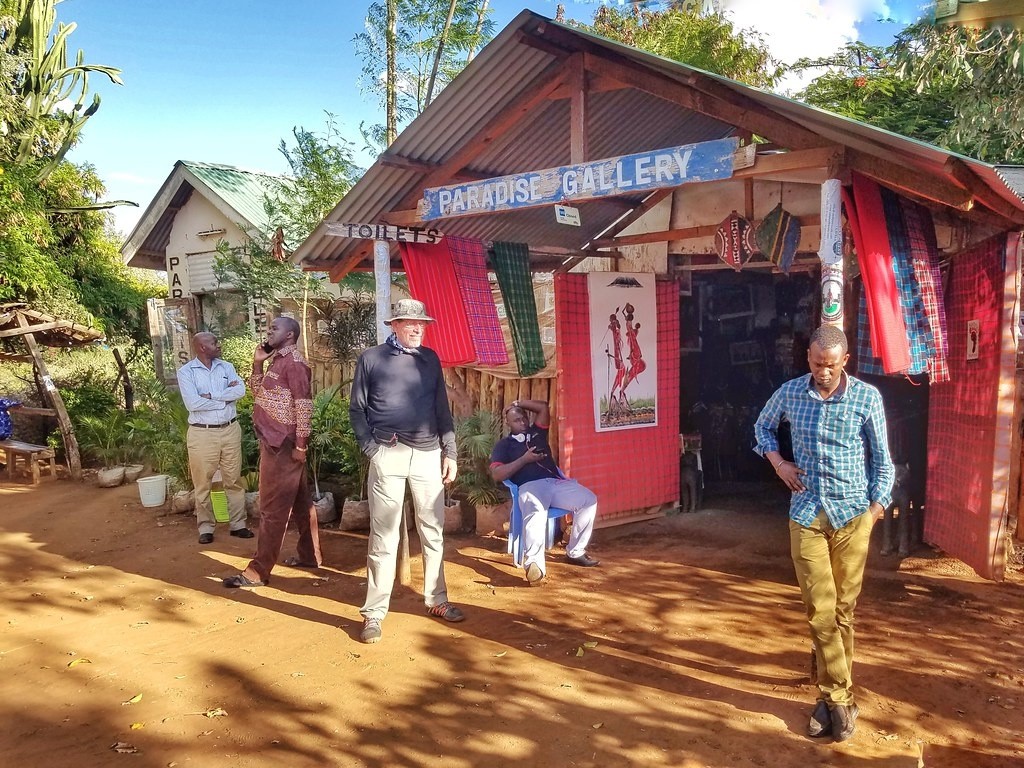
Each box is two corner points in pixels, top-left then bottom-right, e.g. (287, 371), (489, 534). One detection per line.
(136, 475), (168, 508)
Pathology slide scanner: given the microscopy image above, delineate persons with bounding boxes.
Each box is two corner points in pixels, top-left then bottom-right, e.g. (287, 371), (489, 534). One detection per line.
(177, 332), (254, 542)
(349, 298), (466, 644)
(753, 324), (897, 743)
(222, 317), (323, 588)
(0, 399), (23, 471)
(488, 399), (599, 591)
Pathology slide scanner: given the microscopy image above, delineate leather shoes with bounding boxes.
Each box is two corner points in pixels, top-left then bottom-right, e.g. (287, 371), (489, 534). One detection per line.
(807, 700), (832, 737)
(230, 527), (255, 538)
(829, 702), (860, 741)
(198, 531), (213, 544)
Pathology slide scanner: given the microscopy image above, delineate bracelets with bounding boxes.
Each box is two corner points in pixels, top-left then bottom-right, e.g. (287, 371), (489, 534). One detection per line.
(775, 460), (785, 470)
(296, 445), (308, 452)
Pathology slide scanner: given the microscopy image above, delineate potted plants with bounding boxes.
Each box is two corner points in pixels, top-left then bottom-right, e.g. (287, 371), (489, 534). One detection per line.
(45, 366), (504, 534)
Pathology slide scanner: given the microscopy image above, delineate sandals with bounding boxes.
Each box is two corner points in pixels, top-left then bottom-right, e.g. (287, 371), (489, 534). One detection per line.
(283, 557), (321, 567)
(222, 573), (269, 586)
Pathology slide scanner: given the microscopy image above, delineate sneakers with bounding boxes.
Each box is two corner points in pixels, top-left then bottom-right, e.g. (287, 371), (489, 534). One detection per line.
(426, 602), (464, 621)
(360, 617), (382, 643)
(565, 553), (600, 566)
(526, 563), (542, 587)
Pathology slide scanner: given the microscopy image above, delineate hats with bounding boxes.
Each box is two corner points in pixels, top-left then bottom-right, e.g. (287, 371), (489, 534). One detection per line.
(383, 298), (435, 326)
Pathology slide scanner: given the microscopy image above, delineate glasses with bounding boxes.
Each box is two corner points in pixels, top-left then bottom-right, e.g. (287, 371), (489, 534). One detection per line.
(396, 319), (428, 328)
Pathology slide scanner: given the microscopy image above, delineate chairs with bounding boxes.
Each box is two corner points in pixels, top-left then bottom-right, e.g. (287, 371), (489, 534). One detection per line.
(502, 465), (574, 568)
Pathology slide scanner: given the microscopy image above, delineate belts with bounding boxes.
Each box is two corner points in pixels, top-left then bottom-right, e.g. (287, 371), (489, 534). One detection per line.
(192, 418), (237, 429)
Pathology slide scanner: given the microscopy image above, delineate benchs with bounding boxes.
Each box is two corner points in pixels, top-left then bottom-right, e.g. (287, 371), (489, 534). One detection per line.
(0, 437), (53, 484)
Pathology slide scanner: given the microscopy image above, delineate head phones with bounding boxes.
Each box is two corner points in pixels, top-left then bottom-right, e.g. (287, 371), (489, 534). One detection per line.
(509, 428), (532, 443)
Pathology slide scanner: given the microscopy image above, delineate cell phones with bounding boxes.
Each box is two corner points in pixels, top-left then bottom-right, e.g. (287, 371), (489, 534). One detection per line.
(536, 449), (546, 455)
(264, 342), (274, 354)
